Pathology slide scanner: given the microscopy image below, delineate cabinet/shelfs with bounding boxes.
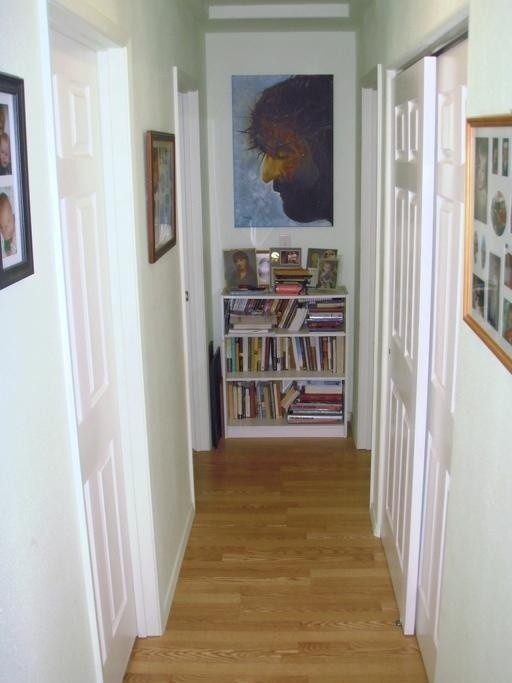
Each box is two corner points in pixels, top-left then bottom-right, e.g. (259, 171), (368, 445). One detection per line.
(222, 295), (346, 439)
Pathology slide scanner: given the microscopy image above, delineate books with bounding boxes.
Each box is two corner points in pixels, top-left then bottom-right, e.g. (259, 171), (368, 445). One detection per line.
(224, 298), (343, 425)
(273, 266), (313, 295)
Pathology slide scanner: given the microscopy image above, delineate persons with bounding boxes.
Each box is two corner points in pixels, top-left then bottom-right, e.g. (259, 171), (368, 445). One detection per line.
(231, 251), (257, 288)
(287, 251), (298, 264)
(0, 132), (12, 176)
(0, 191), (17, 259)
(317, 260), (336, 288)
(236, 75), (333, 227)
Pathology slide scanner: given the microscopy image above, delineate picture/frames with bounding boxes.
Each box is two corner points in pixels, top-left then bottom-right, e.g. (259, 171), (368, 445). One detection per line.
(319, 259), (339, 288)
(256, 252), (271, 288)
(224, 248), (256, 290)
(307, 248), (337, 288)
(269, 247), (302, 290)
(463, 114), (511, 372)
(143, 129), (175, 264)
(0, 72), (36, 291)
